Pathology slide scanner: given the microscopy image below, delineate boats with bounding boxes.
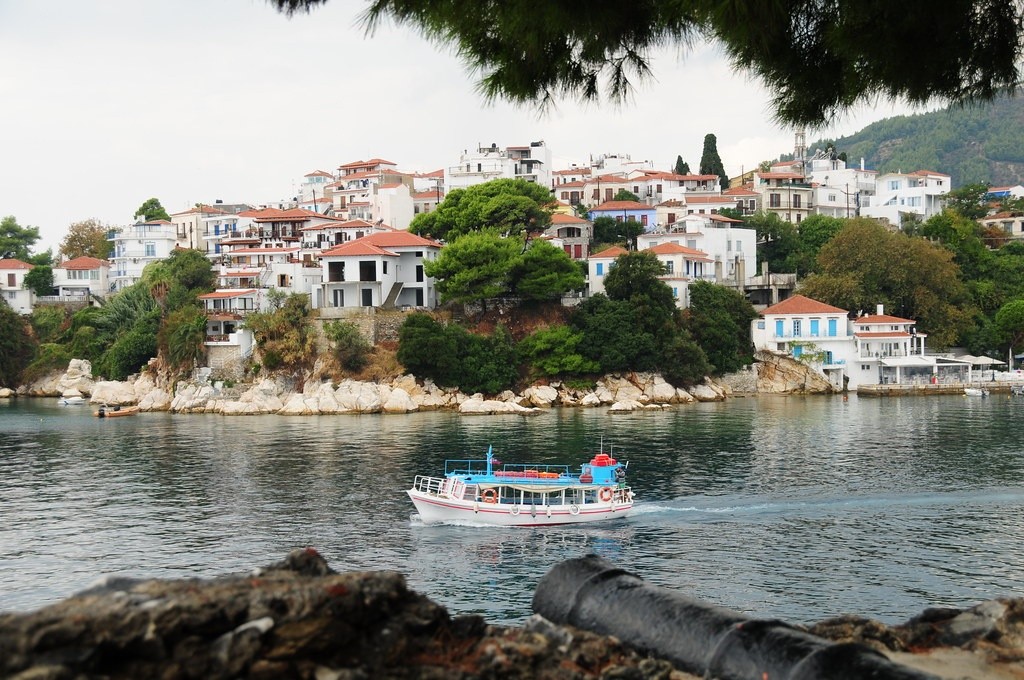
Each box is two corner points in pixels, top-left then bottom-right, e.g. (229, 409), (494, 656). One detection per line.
(95, 405), (140, 417)
(963, 387), (990, 397)
(405, 443), (636, 526)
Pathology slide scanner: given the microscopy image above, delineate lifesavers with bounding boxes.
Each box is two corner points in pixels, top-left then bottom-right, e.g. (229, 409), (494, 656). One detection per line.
(600, 487), (613, 501)
(569, 504), (580, 515)
(482, 489), (498, 503)
(510, 506), (519, 515)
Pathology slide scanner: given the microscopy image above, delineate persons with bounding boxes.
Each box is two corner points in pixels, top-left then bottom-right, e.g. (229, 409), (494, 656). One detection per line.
(615, 466), (625, 500)
